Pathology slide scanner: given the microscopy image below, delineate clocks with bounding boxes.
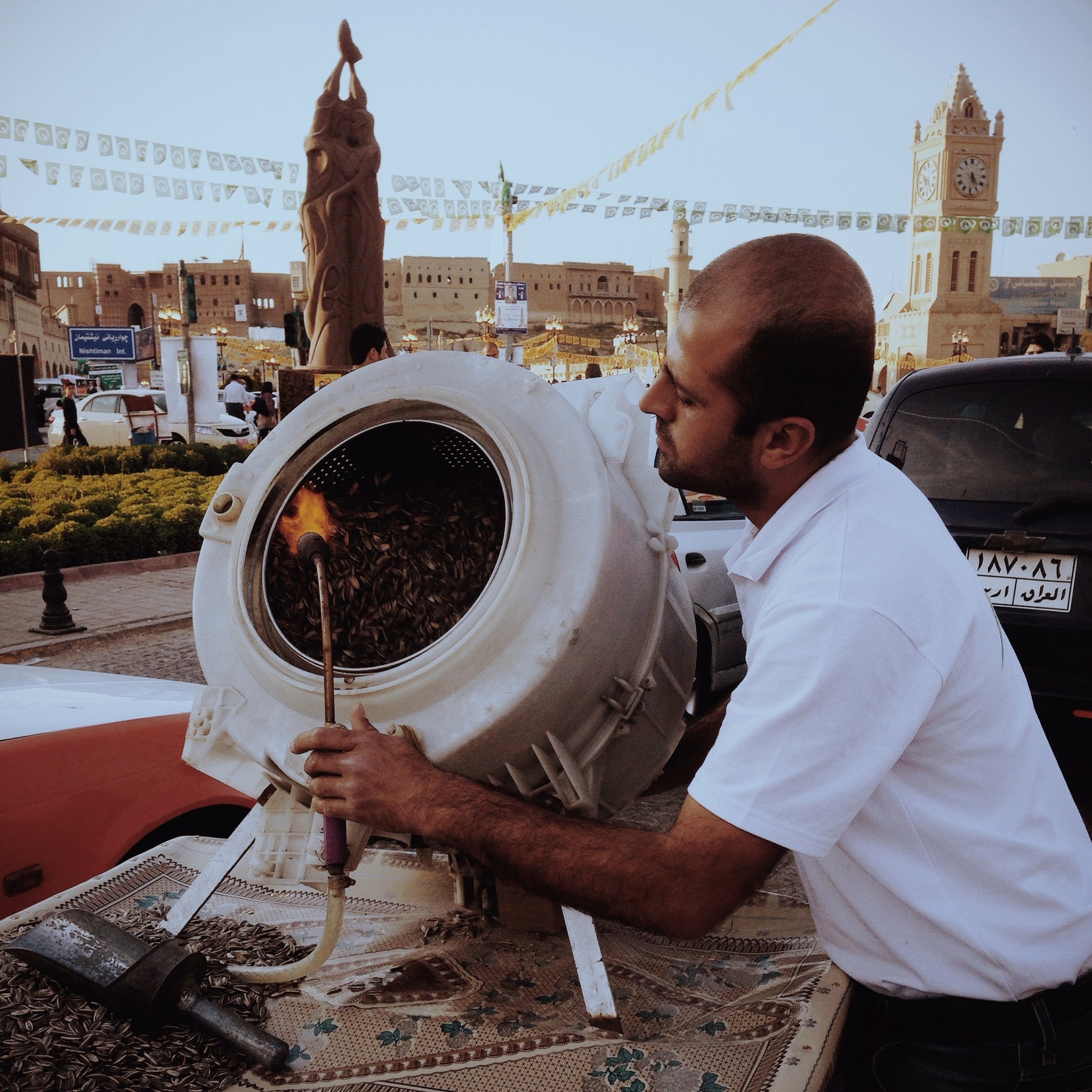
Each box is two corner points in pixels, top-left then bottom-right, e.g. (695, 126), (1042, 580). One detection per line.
(955, 157), (988, 195)
(917, 159), (937, 199)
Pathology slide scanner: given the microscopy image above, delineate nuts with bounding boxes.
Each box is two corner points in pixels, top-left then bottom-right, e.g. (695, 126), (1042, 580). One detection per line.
(0, 902), (502, 1092)
(265, 469), (505, 669)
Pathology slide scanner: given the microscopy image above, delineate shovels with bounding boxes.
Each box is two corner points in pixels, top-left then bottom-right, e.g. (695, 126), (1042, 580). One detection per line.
(2, 909), (290, 1070)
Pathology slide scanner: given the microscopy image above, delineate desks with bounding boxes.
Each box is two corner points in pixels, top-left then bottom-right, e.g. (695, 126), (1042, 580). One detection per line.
(0, 836), (854, 1092)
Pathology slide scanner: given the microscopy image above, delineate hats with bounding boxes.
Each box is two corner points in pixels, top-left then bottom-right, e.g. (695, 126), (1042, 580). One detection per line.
(262, 381), (274, 391)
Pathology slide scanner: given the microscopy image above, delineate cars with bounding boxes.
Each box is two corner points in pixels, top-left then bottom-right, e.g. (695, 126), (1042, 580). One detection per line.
(47, 389), (258, 451)
(665, 428), (864, 729)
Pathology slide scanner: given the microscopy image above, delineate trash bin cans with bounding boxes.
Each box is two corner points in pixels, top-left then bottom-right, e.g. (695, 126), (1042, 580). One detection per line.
(132, 427), (156, 446)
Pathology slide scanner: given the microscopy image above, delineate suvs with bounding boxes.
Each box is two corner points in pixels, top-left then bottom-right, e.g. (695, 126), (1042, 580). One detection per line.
(865, 327), (1092, 838)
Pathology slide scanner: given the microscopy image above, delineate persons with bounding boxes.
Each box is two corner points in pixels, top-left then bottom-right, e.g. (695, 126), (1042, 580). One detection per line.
(258, 381), (278, 441)
(482, 342), (499, 359)
(218, 358), (227, 371)
(94, 375), (107, 393)
(349, 322), (389, 372)
(223, 374), (250, 422)
(1025, 332), (1053, 356)
(87, 381), (97, 394)
(290, 232), (1092, 1092)
(62, 383), (89, 447)
(34, 383), (62, 428)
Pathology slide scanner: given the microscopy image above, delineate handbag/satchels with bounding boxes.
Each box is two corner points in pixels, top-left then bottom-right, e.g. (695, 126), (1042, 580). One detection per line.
(251, 396), (270, 417)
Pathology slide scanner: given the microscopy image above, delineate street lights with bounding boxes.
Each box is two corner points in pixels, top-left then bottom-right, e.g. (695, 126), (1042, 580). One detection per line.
(869, 338), (882, 392)
(475, 305), (495, 343)
(157, 302), (182, 337)
(545, 314), (563, 383)
(953, 329), (969, 364)
(265, 357), (280, 387)
(623, 316), (639, 373)
(255, 343), (270, 383)
(211, 322), (228, 384)
(402, 329), (418, 354)
